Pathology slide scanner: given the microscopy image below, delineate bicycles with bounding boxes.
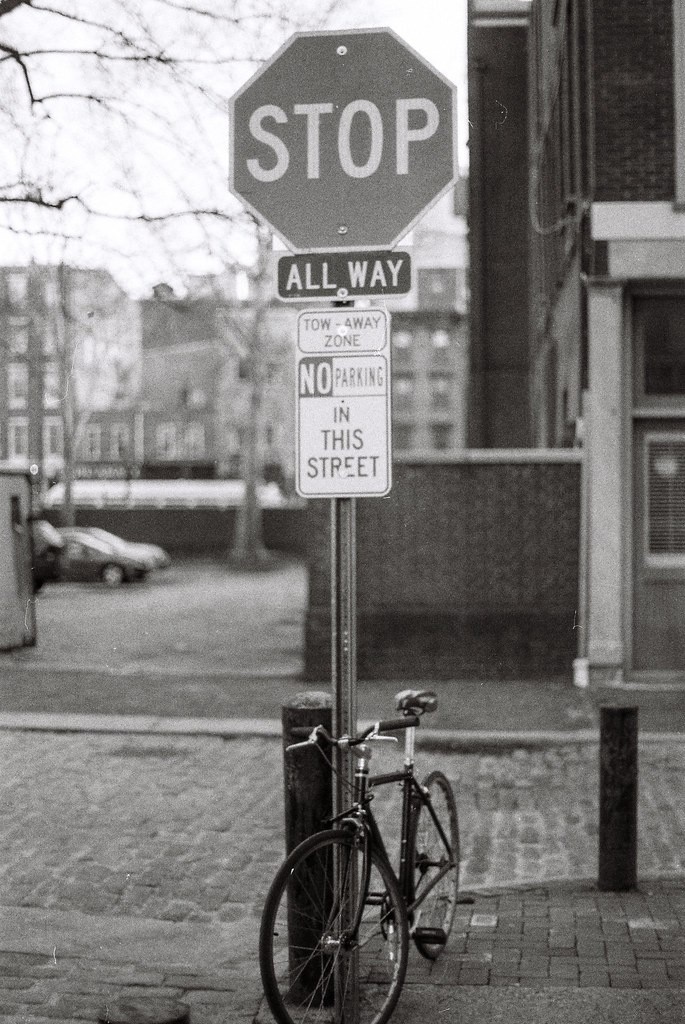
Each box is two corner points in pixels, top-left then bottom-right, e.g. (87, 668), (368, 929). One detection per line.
(257, 688), (461, 1024)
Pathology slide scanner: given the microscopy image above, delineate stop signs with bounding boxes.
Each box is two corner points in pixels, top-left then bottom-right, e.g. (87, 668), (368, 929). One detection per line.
(228, 28), (460, 260)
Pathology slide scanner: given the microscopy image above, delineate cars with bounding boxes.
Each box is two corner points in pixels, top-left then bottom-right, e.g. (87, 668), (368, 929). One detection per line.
(47, 526), (166, 589)
